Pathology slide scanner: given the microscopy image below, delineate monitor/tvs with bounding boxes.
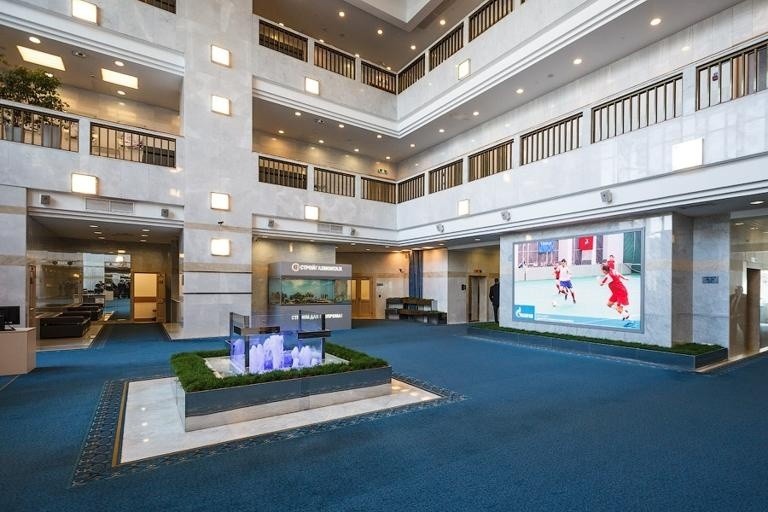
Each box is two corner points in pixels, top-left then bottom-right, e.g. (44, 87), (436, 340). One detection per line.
(0, 306), (20, 331)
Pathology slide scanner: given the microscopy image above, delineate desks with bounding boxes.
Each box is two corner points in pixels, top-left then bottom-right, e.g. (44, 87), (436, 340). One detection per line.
(0, 327), (36, 376)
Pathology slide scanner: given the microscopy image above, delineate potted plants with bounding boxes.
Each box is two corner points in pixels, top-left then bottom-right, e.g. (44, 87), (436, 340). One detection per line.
(0, 65), (70, 150)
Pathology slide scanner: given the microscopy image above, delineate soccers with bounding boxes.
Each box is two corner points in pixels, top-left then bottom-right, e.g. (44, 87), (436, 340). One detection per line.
(552, 300), (559, 308)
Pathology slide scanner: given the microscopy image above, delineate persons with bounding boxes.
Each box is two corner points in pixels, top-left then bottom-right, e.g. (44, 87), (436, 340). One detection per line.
(601, 265), (629, 320)
(110, 278), (131, 300)
(551, 262), (562, 294)
(554, 259), (577, 304)
(488, 277), (499, 323)
(599, 259), (628, 285)
(729, 285), (747, 354)
(607, 255), (617, 271)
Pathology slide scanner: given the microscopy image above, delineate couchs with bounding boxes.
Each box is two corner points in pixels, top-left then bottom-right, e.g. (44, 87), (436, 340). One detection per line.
(40, 303), (105, 339)
